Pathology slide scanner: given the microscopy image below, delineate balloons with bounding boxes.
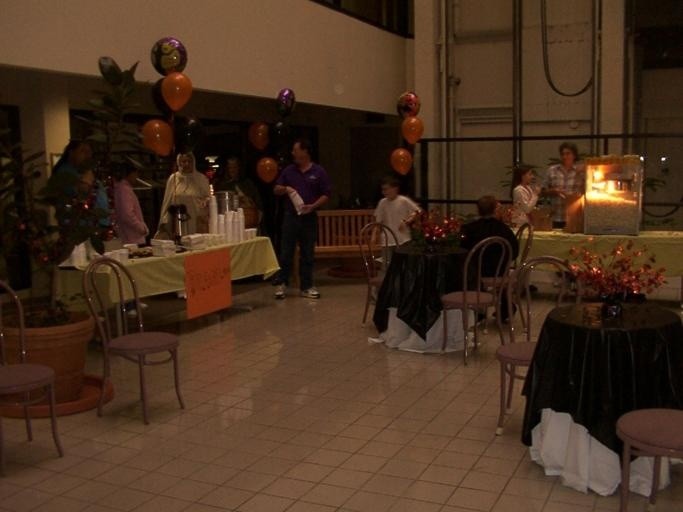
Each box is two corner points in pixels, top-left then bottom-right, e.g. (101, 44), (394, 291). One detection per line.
(277, 88), (296, 118)
(256, 156), (278, 185)
(390, 147), (412, 176)
(249, 121), (272, 152)
(160, 72), (192, 111)
(401, 115), (423, 145)
(142, 119), (173, 157)
(396, 91), (420, 118)
(150, 36), (188, 78)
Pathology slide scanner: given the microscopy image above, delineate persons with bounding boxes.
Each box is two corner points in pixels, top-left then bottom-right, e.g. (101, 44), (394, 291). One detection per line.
(369, 177), (422, 305)
(214, 154), (265, 235)
(539, 142), (585, 228)
(153, 150), (210, 299)
(110, 155), (149, 320)
(459, 195), (520, 329)
(509, 165), (542, 226)
(48, 138), (112, 256)
(272, 140), (332, 299)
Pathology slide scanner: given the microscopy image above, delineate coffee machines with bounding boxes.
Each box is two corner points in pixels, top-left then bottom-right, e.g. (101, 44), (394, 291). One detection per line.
(168, 204), (189, 249)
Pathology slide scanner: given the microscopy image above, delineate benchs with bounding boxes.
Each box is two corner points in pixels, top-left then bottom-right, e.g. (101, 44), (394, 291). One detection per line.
(293, 208), (381, 283)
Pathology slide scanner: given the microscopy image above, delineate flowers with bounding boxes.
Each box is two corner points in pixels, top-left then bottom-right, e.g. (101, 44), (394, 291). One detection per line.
(564, 236), (666, 299)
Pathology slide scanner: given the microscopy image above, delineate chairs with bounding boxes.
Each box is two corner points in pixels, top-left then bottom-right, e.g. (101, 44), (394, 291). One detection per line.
(84, 256), (186, 426)
(0, 281), (65, 457)
(358, 222), (585, 436)
(616, 407), (683, 512)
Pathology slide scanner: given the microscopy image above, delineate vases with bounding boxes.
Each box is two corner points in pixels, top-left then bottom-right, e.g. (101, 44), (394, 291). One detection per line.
(601, 296), (622, 321)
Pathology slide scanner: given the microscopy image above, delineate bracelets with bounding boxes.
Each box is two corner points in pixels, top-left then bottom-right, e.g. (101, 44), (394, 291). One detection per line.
(402, 219), (409, 226)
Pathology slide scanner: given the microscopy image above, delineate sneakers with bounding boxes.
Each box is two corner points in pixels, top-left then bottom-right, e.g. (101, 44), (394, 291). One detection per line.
(275, 284), (286, 298)
(302, 288), (320, 298)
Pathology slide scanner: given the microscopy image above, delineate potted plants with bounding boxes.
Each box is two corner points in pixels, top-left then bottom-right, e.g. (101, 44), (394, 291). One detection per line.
(329, 193), (382, 272)
(0, 56), (151, 418)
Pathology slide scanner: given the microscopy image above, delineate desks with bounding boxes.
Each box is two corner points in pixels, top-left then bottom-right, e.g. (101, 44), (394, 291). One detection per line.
(511, 233), (683, 297)
(52, 237), (281, 346)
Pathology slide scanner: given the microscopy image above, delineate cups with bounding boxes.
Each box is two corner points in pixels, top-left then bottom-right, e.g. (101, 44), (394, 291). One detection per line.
(103, 248), (128, 266)
(209, 193), (256, 243)
